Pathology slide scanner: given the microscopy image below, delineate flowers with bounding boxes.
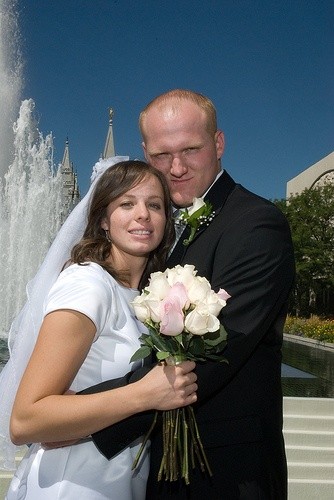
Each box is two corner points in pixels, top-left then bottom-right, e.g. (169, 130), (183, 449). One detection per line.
(173, 195), (216, 245)
(131, 265), (232, 486)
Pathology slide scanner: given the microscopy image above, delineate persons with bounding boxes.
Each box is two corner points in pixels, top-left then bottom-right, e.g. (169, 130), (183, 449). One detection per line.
(26, 88), (296, 500)
(1, 155), (198, 500)
